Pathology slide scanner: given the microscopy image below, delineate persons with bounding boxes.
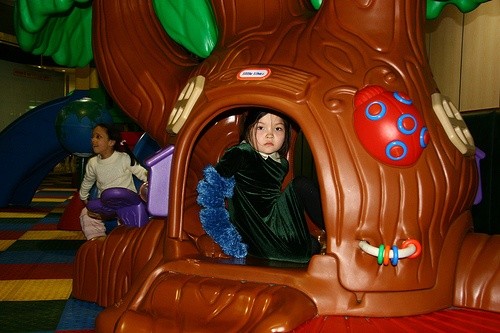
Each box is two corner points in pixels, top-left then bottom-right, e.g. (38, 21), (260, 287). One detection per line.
(78, 124), (149, 240)
(197, 108), (328, 265)
